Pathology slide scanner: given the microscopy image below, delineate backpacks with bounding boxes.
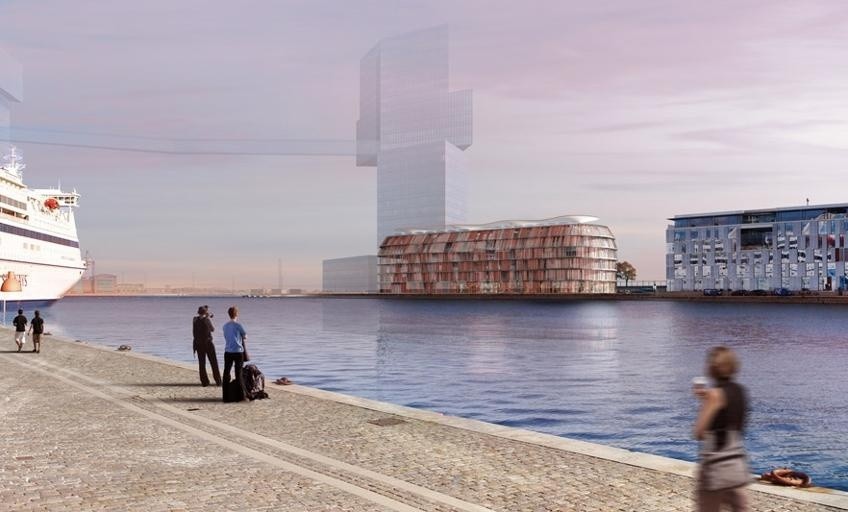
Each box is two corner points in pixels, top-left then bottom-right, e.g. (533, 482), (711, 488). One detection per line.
(243, 364), (269, 402)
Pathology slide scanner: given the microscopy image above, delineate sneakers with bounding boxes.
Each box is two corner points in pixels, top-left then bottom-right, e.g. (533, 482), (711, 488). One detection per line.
(202, 378), (249, 403)
(17, 345), (41, 354)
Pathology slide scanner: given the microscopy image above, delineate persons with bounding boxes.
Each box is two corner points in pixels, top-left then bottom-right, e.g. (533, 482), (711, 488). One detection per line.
(690, 347), (755, 510)
(13, 309), (28, 350)
(221, 306), (251, 401)
(192, 305), (222, 386)
(27, 310), (44, 353)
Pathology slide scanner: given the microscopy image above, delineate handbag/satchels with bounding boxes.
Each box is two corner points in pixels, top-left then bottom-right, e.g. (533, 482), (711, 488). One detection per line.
(193, 339), (196, 354)
(701, 448), (750, 491)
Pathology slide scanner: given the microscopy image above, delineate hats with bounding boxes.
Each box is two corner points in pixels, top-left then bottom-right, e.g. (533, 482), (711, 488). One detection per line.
(198, 304), (209, 314)
